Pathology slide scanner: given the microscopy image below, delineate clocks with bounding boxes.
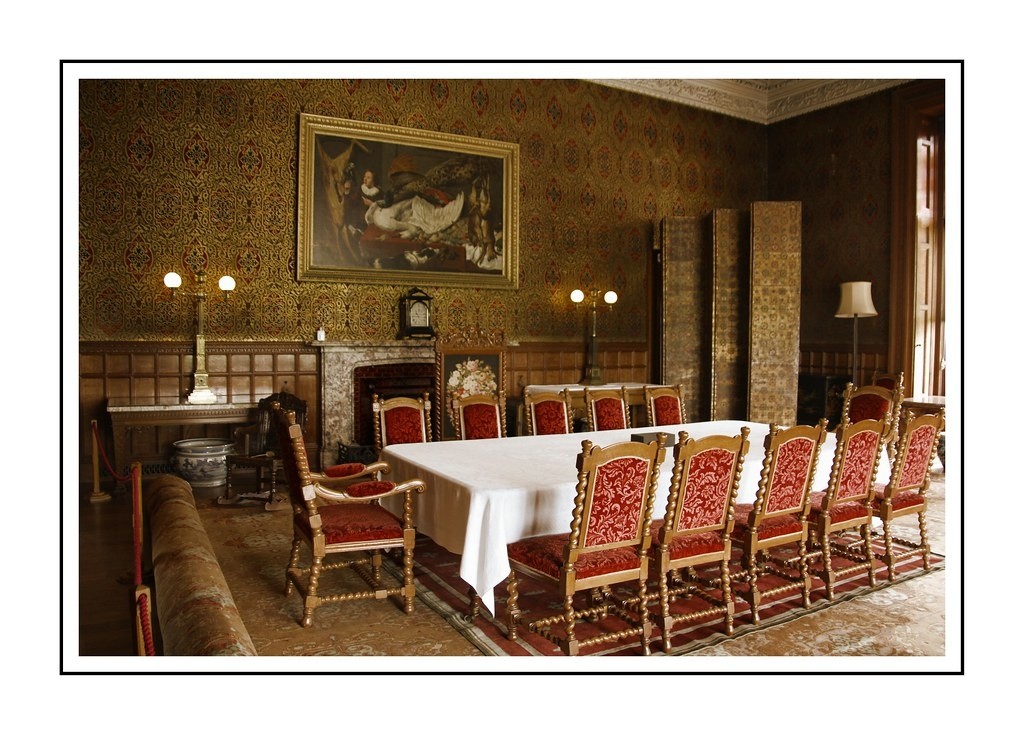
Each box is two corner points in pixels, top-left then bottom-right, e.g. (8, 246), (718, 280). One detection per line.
(395, 287), (437, 343)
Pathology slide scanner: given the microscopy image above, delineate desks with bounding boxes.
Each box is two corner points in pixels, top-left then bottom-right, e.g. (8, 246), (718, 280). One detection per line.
(105, 396), (265, 500)
(525, 382), (683, 431)
(378, 420), (943, 621)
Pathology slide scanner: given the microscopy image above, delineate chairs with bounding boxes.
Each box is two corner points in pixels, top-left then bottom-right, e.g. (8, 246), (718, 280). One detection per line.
(371, 392), (432, 460)
(819, 407), (946, 582)
(643, 384), (687, 427)
(216, 391), (310, 510)
(583, 385), (631, 433)
(450, 390), (507, 441)
(523, 388), (574, 437)
(602, 425), (752, 652)
(831, 371), (905, 473)
(267, 400), (427, 629)
(683, 417), (830, 628)
(506, 431), (668, 656)
(755, 411), (891, 600)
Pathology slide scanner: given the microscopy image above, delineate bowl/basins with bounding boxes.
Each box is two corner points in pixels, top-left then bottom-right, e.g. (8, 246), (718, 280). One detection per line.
(172, 438), (235, 488)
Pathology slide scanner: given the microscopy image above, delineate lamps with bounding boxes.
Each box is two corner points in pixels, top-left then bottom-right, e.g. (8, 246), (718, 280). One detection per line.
(570, 286), (619, 385)
(164, 269), (236, 405)
(835, 281), (878, 389)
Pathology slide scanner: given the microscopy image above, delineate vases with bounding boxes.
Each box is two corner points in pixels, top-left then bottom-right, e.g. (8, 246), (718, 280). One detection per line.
(171, 438), (238, 488)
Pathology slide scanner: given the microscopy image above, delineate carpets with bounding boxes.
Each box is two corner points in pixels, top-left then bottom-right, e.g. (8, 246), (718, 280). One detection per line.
(366, 527), (946, 655)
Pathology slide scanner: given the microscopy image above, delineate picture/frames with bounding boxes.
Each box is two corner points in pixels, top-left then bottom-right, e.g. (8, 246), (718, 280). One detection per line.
(296, 112), (520, 290)
